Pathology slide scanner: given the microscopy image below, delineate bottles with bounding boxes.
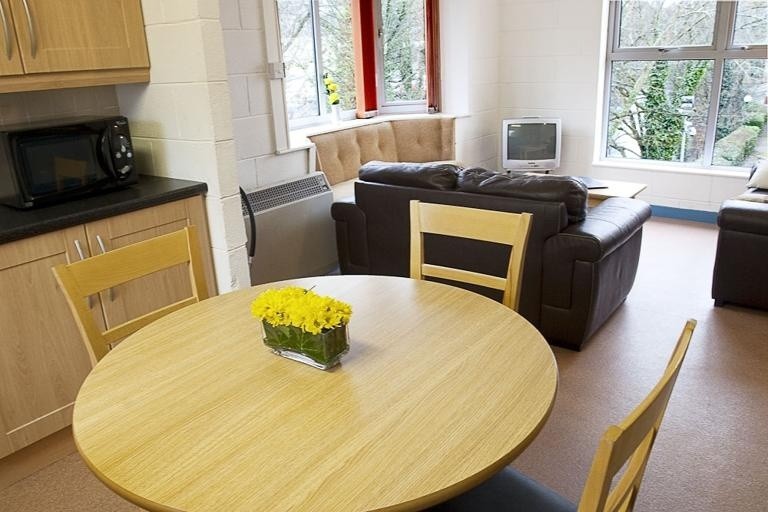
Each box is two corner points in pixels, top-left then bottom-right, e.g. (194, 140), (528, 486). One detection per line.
(332, 102), (343, 127)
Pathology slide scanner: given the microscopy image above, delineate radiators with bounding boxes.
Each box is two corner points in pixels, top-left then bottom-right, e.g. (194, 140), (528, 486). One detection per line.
(240, 172), (340, 286)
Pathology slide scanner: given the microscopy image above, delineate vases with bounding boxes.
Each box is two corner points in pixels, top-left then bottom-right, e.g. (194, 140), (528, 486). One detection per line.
(330, 104), (343, 124)
(261, 317), (350, 364)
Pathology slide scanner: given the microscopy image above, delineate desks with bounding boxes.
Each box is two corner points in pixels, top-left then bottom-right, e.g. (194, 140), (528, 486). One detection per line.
(71, 275), (559, 512)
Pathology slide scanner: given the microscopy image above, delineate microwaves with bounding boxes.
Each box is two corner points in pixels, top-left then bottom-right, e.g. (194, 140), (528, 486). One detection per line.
(0, 114), (139, 211)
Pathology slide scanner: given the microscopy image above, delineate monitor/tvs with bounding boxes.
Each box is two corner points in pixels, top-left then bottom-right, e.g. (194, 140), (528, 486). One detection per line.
(503, 118), (561, 171)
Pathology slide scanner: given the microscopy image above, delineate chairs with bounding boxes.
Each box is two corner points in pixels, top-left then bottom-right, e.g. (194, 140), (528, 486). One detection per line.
(711, 167), (768, 311)
(416, 319), (697, 512)
(50, 225), (210, 369)
(410, 200), (533, 312)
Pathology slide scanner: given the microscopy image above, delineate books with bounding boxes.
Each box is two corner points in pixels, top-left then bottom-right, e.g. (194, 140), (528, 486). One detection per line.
(578, 176), (609, 189)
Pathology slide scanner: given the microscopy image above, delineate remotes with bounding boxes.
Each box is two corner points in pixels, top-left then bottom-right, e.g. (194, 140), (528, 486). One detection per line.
(522, 115), (540, 118)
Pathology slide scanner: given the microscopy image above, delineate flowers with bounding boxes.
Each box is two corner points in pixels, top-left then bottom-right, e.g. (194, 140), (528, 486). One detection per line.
(322, 73), (340, 105)
(249, 288), (353, 336)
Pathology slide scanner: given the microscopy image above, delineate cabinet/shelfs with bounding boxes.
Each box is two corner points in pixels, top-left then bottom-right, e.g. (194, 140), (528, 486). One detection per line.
(0, 0), (151, 94)
(0, 195), (219, 460)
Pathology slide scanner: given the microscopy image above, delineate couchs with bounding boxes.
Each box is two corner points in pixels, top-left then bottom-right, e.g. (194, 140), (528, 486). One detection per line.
(331, 180), (652, 352)
(307, 117), (459, 203)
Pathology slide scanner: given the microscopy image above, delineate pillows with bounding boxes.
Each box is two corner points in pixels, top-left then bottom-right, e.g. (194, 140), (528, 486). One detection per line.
(745, 160), (768, 189)
(457, 166), (587, 224)
(358, 159), (465, 190)
